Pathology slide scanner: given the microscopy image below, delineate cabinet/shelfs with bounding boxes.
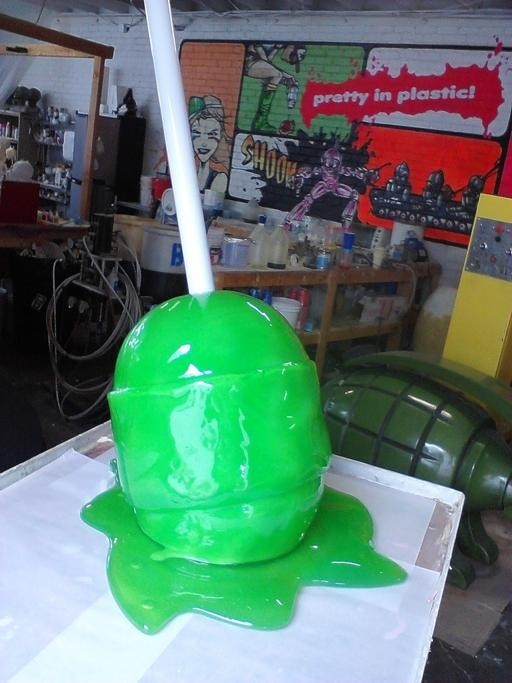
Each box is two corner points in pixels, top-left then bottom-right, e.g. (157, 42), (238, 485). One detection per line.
(0, 107), (43, 169)
(92, 111), (146, 253)
(69, 112), (89, 218)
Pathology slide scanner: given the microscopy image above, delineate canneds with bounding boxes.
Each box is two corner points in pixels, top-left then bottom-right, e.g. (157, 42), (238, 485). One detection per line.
(315, 249), (331, 269)
(288, 287), (309, 331)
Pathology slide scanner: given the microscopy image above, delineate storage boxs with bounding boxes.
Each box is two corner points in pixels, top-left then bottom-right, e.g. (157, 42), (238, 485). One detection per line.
(137, 226), (184, 274)
(111, 213), (160, 263)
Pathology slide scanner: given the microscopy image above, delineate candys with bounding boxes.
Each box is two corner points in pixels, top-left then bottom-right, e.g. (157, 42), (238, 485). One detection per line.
(105, 0), (334, 567)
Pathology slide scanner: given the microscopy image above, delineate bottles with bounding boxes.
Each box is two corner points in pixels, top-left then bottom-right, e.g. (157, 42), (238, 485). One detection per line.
(38, 206), (60, 224)
(43, 104), (71, 124)
(0, 121), (18, 139)
(250, 286), (272, 304)
(246, 213), (331, 271)
(287, 287), (309, 329)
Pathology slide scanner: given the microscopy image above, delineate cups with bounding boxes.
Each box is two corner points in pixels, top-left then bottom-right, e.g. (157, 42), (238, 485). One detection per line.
(343, 230), (356, 249)
(206, 224), (249, 267)
(141, 174), (155, 207)
(336, 246), (354, 268)
(151, 178), (170, 199)
(204, 189), (216, 206)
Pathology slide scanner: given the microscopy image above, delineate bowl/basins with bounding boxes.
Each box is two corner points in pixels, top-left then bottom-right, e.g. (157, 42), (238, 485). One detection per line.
(109, 212), (187, 273)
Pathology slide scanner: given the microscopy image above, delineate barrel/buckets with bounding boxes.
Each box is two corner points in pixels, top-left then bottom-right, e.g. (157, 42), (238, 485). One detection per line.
(271, 294), (304, 330)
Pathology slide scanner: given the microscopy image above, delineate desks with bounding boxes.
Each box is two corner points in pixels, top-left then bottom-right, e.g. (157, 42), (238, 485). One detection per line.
(213, 255), (443, 387)
(1, 218), (89, 251)
